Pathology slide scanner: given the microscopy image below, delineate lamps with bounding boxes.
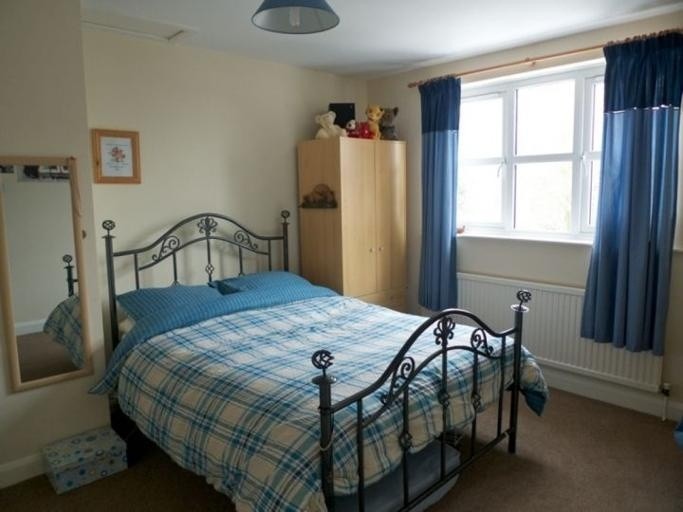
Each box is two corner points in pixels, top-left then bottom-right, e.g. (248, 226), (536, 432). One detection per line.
(250, 0), (340, 35)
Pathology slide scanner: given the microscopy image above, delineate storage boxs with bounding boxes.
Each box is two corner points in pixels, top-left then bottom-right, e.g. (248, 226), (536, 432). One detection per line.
(39, 426), (128, 496)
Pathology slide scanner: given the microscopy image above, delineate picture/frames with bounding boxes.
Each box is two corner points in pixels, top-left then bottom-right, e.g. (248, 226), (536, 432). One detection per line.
(91, 127), (142, 185)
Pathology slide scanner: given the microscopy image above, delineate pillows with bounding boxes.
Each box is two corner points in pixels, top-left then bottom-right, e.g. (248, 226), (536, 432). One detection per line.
(117, 268), (311, 322)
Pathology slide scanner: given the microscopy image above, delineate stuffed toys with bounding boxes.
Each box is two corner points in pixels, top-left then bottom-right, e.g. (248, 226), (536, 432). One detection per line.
(313, 111), (346, 136)
(379, 106), (399, 140)
(344, 118), (370, 138)
(364, 104), (382, 139)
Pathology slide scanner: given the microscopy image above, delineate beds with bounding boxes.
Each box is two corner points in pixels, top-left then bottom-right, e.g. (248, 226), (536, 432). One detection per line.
(102, 210), (530, 511)
(43, 252), (84, 370)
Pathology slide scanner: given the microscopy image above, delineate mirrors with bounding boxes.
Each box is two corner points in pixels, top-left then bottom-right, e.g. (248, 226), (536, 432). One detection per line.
(0, 156), (96, 394)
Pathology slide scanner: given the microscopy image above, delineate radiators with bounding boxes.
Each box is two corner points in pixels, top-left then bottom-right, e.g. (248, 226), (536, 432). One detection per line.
(453, 271), (664, 395)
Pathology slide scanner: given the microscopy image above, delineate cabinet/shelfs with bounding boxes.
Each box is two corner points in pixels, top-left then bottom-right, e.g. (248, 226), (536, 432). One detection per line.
(297, 137), (408, 312)
